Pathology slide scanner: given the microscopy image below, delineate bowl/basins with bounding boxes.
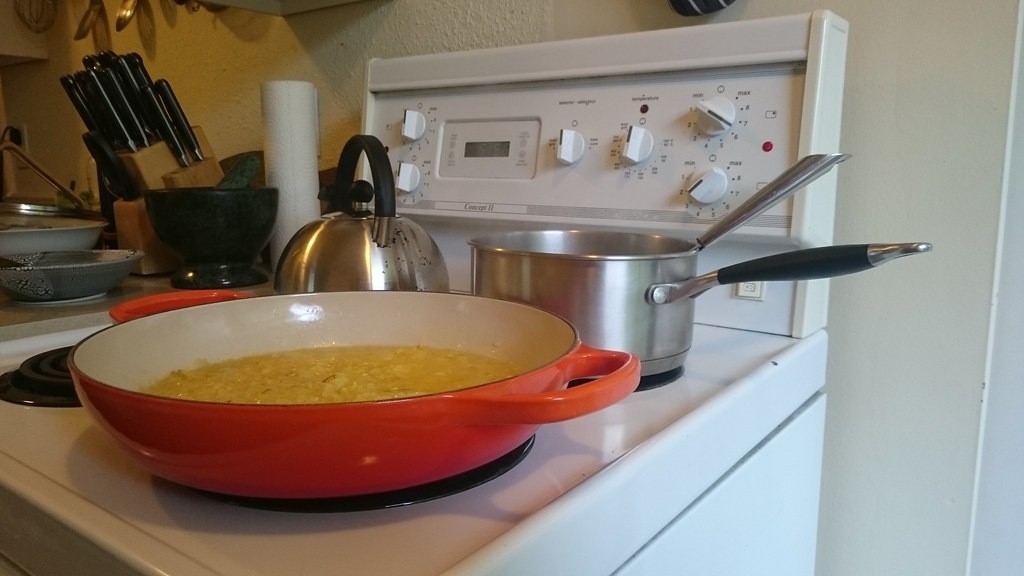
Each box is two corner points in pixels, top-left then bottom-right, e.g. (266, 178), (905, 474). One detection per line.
(0, 214), (110, 258)
(142, 187), (278, 289)
(0, 249), (146, 301)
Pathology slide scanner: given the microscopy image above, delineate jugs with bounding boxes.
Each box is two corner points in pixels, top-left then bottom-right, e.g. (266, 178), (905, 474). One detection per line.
(274, 135), (450, 294)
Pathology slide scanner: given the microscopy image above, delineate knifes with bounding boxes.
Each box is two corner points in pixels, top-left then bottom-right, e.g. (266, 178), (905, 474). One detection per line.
(59, 50), (205, 202)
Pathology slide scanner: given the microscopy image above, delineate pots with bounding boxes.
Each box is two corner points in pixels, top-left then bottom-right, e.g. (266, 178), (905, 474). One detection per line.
(467, 230), (934, 376)
(66, 289), (640, 498)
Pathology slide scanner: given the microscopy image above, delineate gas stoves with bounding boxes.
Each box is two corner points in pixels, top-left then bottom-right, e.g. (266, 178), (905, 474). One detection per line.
(0, 10), (849, 576)
